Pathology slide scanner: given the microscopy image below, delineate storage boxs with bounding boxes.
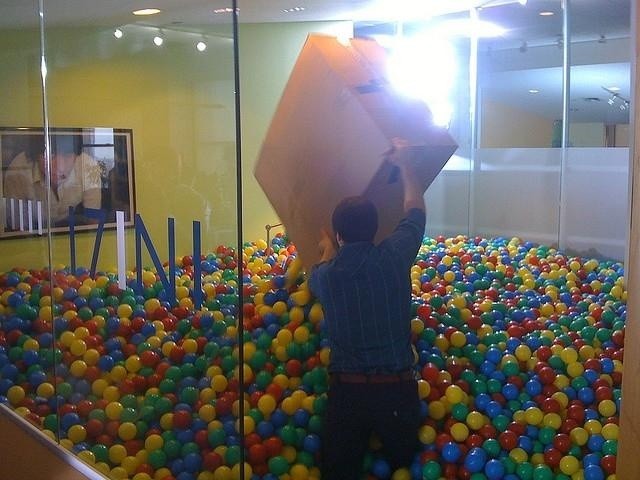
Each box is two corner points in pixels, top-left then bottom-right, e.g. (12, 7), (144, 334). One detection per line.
(251, 31), (460, 276)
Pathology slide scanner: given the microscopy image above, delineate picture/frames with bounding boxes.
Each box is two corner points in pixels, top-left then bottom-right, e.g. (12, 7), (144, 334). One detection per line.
(0, 127), (134, 240)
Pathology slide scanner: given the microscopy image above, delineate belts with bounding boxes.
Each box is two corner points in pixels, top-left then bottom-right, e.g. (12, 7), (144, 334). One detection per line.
(325, 369), (418, 382)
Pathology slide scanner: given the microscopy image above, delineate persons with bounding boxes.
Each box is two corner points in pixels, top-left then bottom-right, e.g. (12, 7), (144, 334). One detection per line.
(4, 128), (102, 230)
(308, 137), (427, 480)
